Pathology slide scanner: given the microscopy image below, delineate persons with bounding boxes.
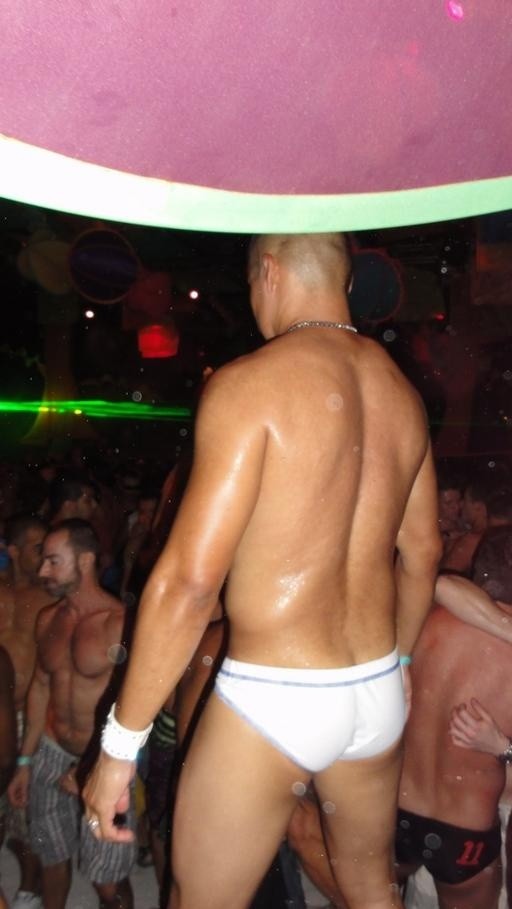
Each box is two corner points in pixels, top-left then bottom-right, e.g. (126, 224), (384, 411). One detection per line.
(2, 429), (510, 908)
(79, 235), (443, 908)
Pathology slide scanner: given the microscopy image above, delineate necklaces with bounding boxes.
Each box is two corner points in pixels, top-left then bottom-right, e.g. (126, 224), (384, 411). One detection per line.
(283, 320), (357, 333)
